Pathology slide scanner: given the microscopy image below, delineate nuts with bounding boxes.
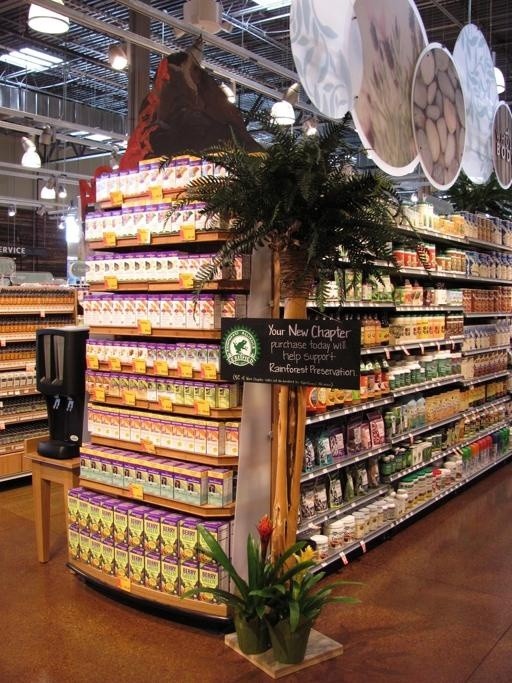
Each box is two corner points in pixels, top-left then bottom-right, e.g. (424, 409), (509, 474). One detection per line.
(411, 49), (466, 185)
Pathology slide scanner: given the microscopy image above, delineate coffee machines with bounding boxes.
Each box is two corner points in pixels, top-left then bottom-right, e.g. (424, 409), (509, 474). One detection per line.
(34, 324), (89, 459)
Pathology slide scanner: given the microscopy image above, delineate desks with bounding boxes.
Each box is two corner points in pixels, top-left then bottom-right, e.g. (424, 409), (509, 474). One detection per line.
(23, 433), (84, 565)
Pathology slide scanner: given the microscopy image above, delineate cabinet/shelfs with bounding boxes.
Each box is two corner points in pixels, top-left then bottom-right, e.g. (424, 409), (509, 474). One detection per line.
(60, 189), (512, 619)
(0, 281), (79, 491)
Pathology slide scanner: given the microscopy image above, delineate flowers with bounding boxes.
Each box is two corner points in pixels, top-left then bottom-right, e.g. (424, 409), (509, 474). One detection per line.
(249, 544), (371, 636)
(189, 509), (304, 621)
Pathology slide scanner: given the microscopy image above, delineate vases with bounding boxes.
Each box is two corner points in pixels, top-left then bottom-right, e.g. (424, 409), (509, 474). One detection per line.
(261, 606), (323, 666)
(230, 603), (272, 654)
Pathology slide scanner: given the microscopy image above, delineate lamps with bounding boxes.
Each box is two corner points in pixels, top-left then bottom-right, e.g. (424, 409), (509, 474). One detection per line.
(115, 140), (127, 147)
(485, 0), (507, 95)
(26, 0), (71, 34)
(40, 175), (55, 199)
(267, 98), (296, 126)
(8, 204), (17, 218)
(108, 42), (128, 70)
(301, 118), (317, 137)
(0, 48), (69, 72)
(20, 135), (41, 169)
(213, 77), (235, 104)
(69, 130), (112, 142)
(109, 148), (120, 170)
(59, 183), (69, 199)
(67, 199), (80, 216)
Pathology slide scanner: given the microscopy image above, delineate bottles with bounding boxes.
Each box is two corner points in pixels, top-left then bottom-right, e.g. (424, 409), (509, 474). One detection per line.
(306, 313), (390, 418)
(461, 212), (511, 471)
(380, 192), (466, 485)
(0, 287), (76, 455)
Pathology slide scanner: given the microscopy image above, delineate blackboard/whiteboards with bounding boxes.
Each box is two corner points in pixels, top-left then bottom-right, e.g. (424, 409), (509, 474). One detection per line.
(221, 318), (361, 390)
